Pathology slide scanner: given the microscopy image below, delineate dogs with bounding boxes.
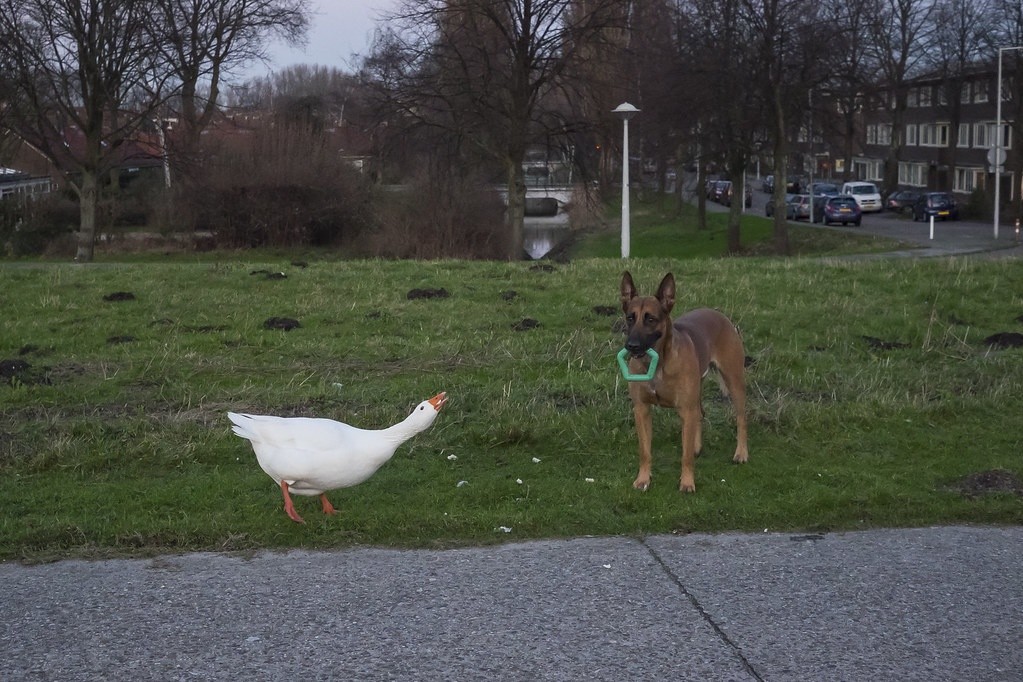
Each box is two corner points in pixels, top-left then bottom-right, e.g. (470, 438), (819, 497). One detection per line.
(620, 270), (750, 496)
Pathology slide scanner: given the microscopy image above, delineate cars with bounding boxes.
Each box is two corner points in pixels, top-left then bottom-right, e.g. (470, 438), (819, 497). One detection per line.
(721, 181), (752, 210)
(813, 184), (841, 203)
(816, 195), (863, 226)
(917, 191), (955, 223)
(763, 193), (793, 218)
(695, 173), (732, 203)
(881, 186), (924, 214)
(782, 196), (814, 221)
(639, 154), (679, 186)
(756, 174), (823, 195)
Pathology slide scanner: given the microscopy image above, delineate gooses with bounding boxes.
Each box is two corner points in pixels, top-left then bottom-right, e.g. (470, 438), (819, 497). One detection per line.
(226, 391), (449, 528)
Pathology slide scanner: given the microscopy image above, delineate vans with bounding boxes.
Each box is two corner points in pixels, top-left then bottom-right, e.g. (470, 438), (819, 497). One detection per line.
(841, 180), (881, 214)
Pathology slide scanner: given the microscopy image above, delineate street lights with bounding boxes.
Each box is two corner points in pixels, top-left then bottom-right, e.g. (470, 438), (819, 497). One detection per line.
(609, 100), (644, 260)
